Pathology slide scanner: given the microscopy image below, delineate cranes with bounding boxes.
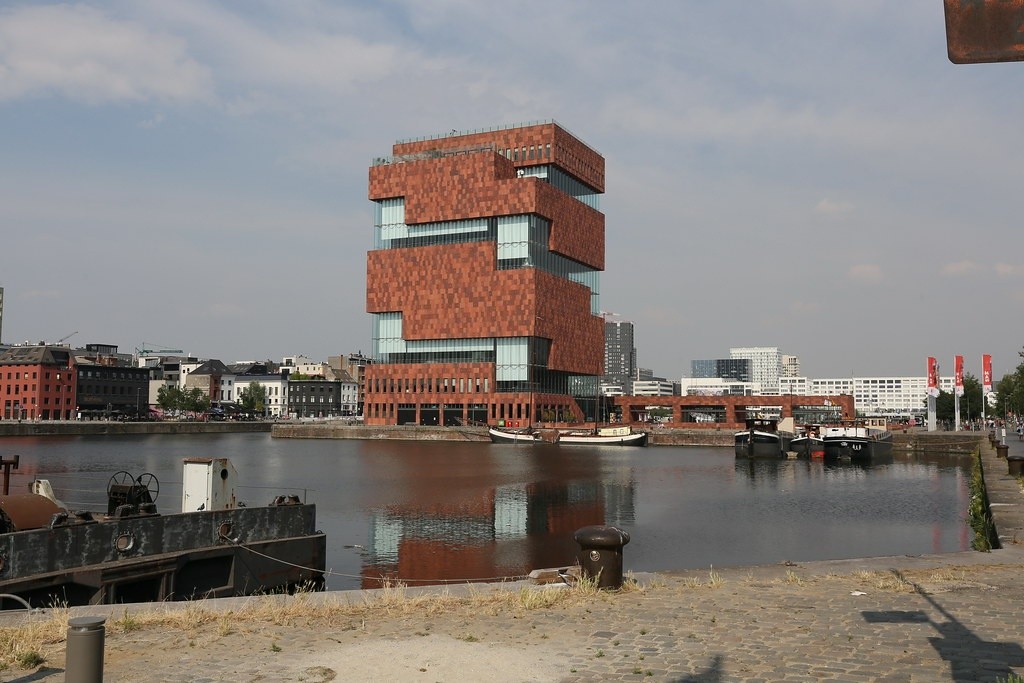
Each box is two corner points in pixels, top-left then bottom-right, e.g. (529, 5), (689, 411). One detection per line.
(135, 341), (183, 358)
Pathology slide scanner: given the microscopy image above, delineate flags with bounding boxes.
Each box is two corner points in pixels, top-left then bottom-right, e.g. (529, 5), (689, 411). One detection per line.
(982, 355), (993, 393)
(928, 357), (940, 397)
(955, 355), (964, 397)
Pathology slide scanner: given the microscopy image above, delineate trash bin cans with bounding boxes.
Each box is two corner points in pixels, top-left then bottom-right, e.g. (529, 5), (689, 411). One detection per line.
(497, 418), (505, 426)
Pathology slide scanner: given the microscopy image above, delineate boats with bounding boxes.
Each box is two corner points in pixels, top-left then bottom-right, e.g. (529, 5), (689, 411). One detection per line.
(0, 455), (327, 611)
(790, 423), (824, 453)
(823, 427), (894, 457)
(735, 418), (794, 459)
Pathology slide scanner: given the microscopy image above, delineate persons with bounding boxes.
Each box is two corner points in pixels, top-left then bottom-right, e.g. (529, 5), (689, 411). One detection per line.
(715, 424), (720, 435)
(964, 415), (1024, 442)
(590, 428), (594, 436)
(650, 423), (653, 431)
(658, 422), (665, 432)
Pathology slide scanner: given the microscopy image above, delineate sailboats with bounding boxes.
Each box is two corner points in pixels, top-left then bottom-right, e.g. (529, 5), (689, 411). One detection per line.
(487, 362), (654, 445)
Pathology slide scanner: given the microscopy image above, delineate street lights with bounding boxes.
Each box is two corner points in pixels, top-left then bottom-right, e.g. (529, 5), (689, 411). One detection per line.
(1005, 398), (1009, 428)
(137, 387), (141, 418)
